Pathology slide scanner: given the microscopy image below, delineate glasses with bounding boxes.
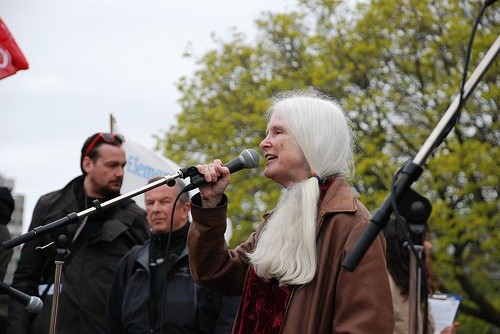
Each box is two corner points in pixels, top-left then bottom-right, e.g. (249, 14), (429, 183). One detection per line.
(84, 132), (122, 158)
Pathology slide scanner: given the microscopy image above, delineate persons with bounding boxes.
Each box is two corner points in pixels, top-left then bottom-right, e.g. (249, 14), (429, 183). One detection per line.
(6, 133), (151, 334)
(386, 212), (460, 334)
(104, 173), (248, 334)
(0, 186), (15, 283)
(187, 88), (396, 334)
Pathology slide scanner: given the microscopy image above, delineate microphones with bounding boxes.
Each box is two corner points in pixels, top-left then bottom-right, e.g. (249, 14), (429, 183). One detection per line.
(0, 281), (44, 314)
(180, 149), (260, 193)
